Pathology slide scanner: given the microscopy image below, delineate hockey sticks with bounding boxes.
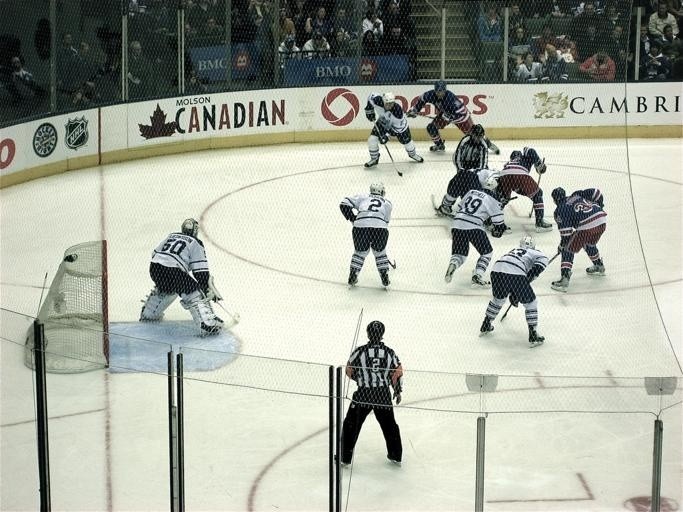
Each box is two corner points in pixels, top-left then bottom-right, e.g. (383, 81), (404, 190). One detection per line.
(509, 196), (517, 200)
(217, 302), (239, 323)
(499, 302), (513, 323)
(418, 112), (435, 120)
(388, 258), (395, 269)
(371, 118), (402, 177)
(528, 157), (545, 218)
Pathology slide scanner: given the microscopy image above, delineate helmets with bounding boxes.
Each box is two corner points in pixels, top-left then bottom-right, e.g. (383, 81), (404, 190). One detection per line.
(370, 184), (384, 195)
(470, 125), (484, 136)
(181, 218), (198, 236)
(520, 236), (537, 250)
(552, 187), (567, 203)
(510, 151), (522, 161)
(436, 80), (446, 91)
(383, 92), (396, 108)
(482, 177), (497, 191)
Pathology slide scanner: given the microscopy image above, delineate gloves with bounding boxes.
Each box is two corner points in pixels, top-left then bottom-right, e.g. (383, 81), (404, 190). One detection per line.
(380, 134), (390, 144)
(367, 112), (377, 121)
(407, 108), (420, 117)
(443, 114), (452, 122)
(536, 164), (547, 174)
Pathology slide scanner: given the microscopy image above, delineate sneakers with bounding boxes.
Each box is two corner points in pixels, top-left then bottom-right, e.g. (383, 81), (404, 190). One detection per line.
(585, 266), (605, 273)
(552, 280), (569, 287)
(199, 319), (224, 333)
(142, 312), (165, 322)
(348, 275), (358, 284)
(529, 335), (544, 341)
(333, 456), (351, 463)
(535, 220), (552, 229)
(409, 153), (423, 162)
(430, 145), (445, 150)
(480, 326), (495, 332)
(445, 265), (455, 282)
(472, 275), (490, 284)
(387, 455), (401, 461)
(487, 141), (499, 156)
(381, 276), (390, 286)
(364, 158), (378, 166)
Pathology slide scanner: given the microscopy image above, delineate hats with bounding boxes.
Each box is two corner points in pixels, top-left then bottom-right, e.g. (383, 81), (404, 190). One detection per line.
(367, 321), (385, 334)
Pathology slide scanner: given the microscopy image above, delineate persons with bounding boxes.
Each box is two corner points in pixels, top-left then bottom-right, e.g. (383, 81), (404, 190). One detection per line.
(57, 31), (84, 67)
(575, 1), (601, 30)
(272, 8), (296, 45)
(7, 56), (49, 102)
(548, 0), (566, 18)
(579, 47), (616, 80)
(136, 0), (267, 37)
(272, 34), (301, 69)
(339, 182), (393, 291)
(311, 8), (333, 37)
(129, 40), (142, 84)
(302, 32), (332, 59)
(510, 27), (530, 60)
(378, 26), (417, 56)
(497, 147), (552, 233)
(364, 93), (423, 169)
(540, 43), (569, 81)
(406, 80), (499, 155)
(445, 176), (505, 289)
(452, 124), (489, 171)
(532, 24), (564, 56)
(551, 187), (607, 293)
(508, 4), (525, 29)
(363, 8), (383, 37)
(335, 30), (350, 58)
(603, 3), (625, 25)
(335, 9), (349, 28)
(362, 30), (378, 57)
(575, 24), (614, 62)
(516, 51), (543, 81)
(479, 237), (549, 348)
(384, 2), (409, 24)
(139, 218), (226, 336)
(334, 320), (403, 466)
(643, 44), (668, 81)
(640, 25), (651, 58)
(649, 2), (680, 41)
(431, 169), (518, 223)
(610, 25), (623, 46)
(478, 2), (503, 42)
(657, 24), (683, 60)
